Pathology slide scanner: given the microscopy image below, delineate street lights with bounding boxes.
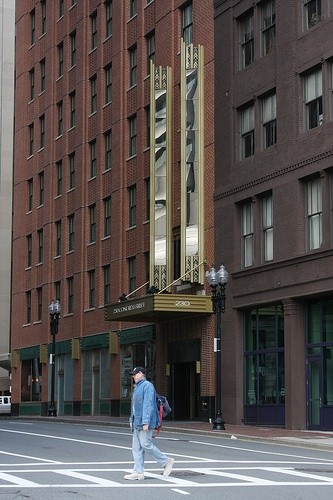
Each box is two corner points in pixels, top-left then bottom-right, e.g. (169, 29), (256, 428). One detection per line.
(47, 299), (61, 416)
(208, 265), (228, 430)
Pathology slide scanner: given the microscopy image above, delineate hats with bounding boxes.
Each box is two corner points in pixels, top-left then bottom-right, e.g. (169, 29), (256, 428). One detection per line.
(129, 367), (146, 376)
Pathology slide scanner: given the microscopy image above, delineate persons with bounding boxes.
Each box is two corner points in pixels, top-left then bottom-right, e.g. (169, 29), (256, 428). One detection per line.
(123, 366), (174, 480)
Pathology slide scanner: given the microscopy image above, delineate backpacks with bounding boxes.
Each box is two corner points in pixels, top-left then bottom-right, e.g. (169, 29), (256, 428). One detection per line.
(152, 394), (172, 438)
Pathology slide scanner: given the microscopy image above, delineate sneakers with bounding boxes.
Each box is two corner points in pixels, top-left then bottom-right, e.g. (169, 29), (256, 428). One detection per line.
(163, 457), (174, 476)
(124, 472), (144, 480)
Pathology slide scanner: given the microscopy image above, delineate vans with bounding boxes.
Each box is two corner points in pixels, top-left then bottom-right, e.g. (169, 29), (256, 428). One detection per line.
(0, 396), (11, 416)
